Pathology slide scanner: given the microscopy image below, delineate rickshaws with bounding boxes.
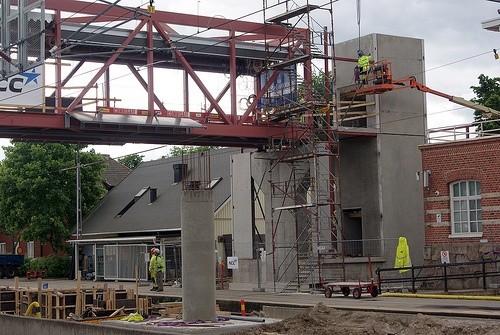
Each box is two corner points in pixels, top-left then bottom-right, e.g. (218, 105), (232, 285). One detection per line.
(315, 248), (380, 300)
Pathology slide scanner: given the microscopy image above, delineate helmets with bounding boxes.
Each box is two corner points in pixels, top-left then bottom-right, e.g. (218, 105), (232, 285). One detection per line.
(153, 248), (160, 255)
(151, 247), (155, 252)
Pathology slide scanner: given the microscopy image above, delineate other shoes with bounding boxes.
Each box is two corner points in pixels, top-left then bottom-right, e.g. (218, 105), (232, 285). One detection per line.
(150, 286), (162, 292)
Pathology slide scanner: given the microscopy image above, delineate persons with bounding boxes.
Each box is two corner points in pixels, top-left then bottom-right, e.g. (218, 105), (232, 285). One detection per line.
(357, 50), (371, 83)
(149, 248), (157, 290)
(154, 248), (165, 292)
